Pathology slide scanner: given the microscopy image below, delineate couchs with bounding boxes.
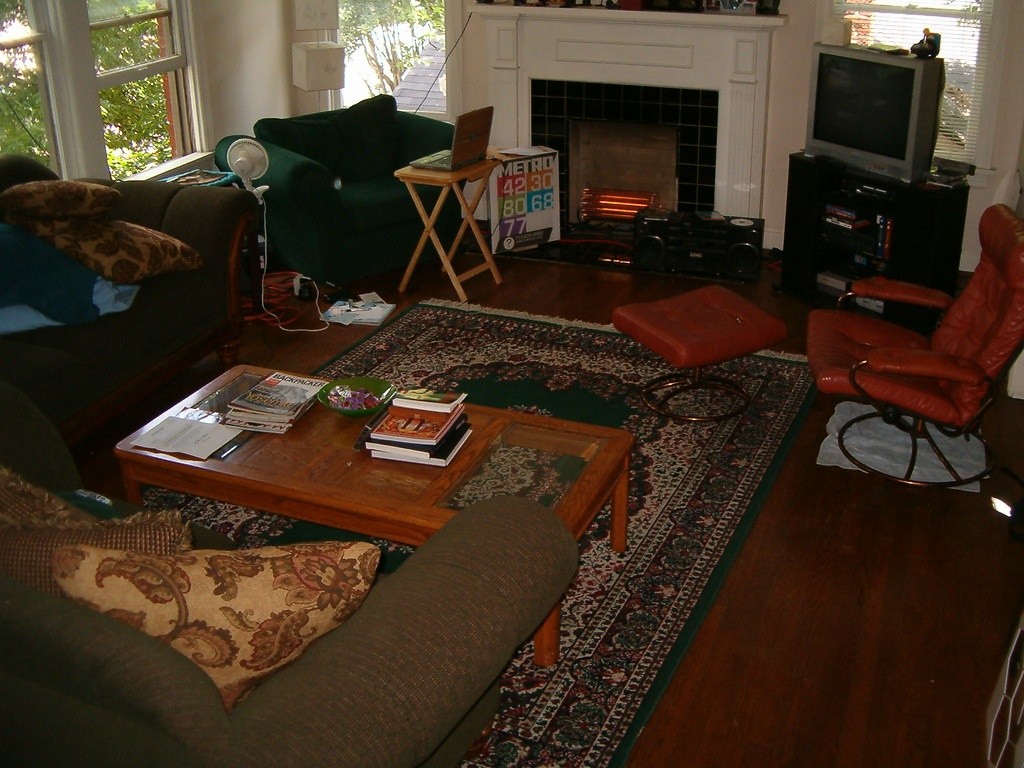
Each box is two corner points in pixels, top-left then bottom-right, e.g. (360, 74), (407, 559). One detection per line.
(0, 154), (263, 447)
(0, 379), (579, 768)
(214, 110), (467, 289)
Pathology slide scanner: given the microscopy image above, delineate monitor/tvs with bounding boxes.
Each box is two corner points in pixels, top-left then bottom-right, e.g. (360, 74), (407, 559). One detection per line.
(805, 41), (945, 182)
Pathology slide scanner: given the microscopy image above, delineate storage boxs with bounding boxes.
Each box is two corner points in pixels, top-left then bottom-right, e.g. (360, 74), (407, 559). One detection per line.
(486, 145), (561, 253)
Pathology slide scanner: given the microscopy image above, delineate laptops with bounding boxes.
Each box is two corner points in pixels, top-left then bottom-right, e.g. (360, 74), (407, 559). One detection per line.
(409, 105), (494, 172)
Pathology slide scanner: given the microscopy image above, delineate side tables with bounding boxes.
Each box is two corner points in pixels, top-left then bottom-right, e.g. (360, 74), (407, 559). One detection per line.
(171, 169), (261, 311)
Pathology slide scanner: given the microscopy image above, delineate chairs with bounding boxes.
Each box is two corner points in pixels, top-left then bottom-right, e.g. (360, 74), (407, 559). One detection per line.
(807, 203), (1024, 490)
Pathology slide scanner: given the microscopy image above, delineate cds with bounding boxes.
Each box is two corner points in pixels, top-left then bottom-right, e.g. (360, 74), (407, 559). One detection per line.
(730, 218), (754, 226)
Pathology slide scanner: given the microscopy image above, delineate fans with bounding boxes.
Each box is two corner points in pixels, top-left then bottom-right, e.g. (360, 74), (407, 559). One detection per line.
(226, 137), (270, 206)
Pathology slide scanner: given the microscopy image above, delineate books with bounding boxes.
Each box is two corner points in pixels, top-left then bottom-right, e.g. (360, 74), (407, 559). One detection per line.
(830, 205), (869, 229)
(365, 386), (472, 466)
(875, 216), (891, 260)
(216, 371), (330, 435)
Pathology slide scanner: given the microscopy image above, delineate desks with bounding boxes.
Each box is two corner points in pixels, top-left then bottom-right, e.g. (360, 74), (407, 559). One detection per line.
(395, 153), (502, 303)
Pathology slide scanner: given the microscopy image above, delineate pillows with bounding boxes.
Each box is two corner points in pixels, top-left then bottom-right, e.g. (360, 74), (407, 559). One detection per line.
(0, 512), (193, 592)
(0, 224), (139, 336)
(28, 219), (203, 281)
(49, 538), (381, 708)
(0, 466), (101, 529)
(254, 94), (399, 179)
(0, 180), (121, 217)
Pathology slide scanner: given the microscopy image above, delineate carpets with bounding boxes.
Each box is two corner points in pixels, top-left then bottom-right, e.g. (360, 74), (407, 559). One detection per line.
(144, 297), (819, 768)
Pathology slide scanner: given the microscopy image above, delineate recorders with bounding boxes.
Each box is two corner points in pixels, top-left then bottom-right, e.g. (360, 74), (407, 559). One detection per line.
(632, 205), (765, 279)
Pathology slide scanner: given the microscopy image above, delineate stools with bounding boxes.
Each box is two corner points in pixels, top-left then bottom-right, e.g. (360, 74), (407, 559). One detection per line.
(612, 283), (788, 422)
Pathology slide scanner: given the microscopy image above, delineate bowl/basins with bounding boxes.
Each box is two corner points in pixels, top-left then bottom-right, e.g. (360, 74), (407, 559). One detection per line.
(317, 376), (397, 421)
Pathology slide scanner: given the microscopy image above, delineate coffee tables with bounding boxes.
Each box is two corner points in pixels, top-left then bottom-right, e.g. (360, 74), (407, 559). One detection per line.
(115, 362), (633, 666)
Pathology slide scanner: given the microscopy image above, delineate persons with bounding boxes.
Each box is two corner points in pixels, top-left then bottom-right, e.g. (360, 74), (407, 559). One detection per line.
(322, 300), (397, 327)
(398, 387), (459, 402)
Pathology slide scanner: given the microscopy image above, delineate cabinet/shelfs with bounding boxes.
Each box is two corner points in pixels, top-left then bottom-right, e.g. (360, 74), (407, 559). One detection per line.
(782, 153), (971, 331)
